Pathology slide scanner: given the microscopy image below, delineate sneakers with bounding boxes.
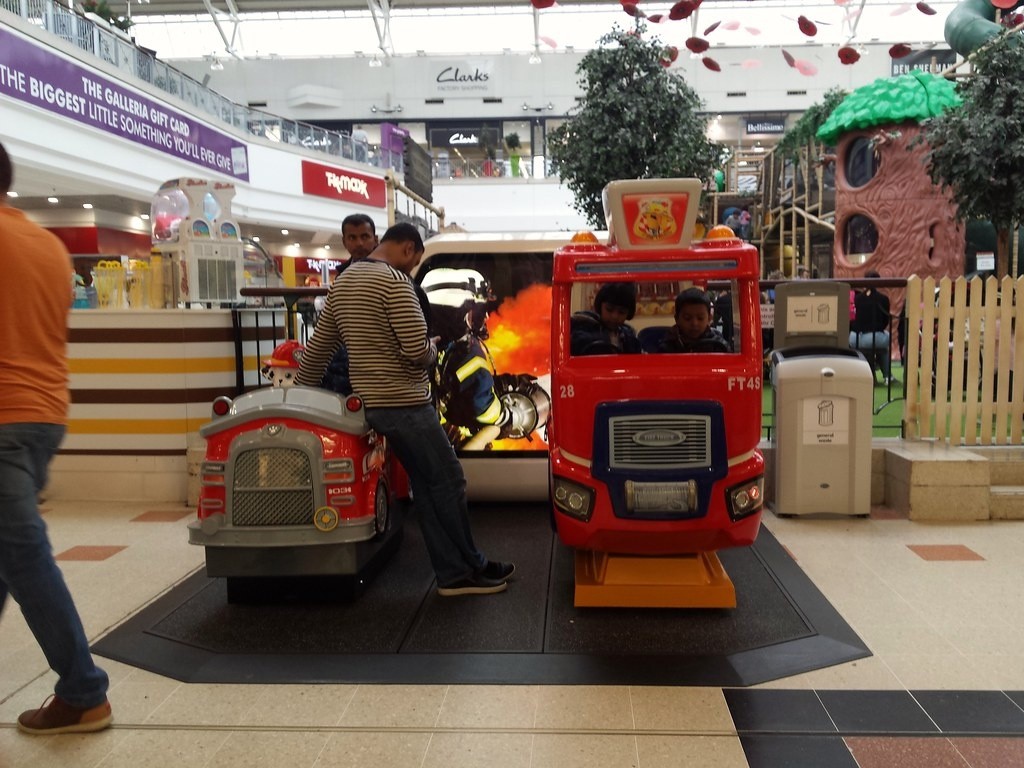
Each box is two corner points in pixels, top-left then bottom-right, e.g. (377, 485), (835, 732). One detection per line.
(438, 572), (508, 597)
(477, 560), (515, 583)
(17, 694), (112, 735)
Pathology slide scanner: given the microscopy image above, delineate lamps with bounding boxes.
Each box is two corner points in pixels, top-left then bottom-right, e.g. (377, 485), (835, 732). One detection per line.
(856, 40), (868, 57)
(369, 53), (381, 67)
(211, 55), (223, 70)
(528, 51), (541, 65)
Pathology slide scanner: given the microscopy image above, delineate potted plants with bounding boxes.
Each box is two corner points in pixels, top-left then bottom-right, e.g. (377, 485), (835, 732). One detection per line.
(505, 133), (523, 176)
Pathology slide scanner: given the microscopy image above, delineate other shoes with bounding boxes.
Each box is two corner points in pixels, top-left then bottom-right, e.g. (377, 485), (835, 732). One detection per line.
(885, 377), (900, 385)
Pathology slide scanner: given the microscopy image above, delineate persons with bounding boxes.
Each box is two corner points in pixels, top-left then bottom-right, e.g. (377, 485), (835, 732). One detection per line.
(850, 269), (896, 386)
(294, 222), (515, 595)
(898, 285), (1024, 398)
(320, 214), (432, 397)
(725, 210), (750, 239)
(571, 282), (638, 357)
(799, 269), (811, 279)
(631, 287), (733, 353)
(0, 144), (112, 736)
(760, 289), (775, 304)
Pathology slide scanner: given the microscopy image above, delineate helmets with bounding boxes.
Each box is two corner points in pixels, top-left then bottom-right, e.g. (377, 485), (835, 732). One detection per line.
(419, 268), (495, 342)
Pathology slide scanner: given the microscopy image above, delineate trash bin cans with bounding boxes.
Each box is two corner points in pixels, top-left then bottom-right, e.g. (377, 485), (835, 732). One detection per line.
(769, 344), (875, 521)
